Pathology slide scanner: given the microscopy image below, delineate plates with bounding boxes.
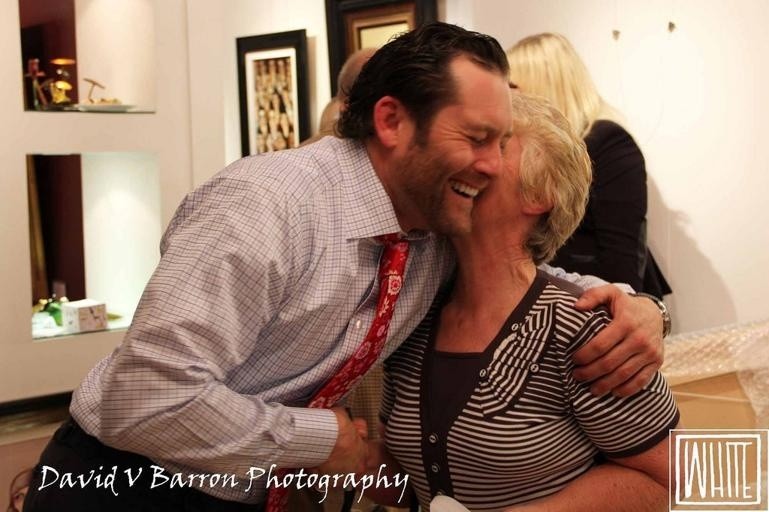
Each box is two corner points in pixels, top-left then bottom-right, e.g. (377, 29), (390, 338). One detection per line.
(73, 103), (137, 112)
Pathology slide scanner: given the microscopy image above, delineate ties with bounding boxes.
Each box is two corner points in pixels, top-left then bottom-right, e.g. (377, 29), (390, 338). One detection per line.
(267, 233), (411, 512)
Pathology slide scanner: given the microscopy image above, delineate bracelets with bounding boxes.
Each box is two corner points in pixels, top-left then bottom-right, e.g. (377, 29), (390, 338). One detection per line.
(629, 291), (671, 338)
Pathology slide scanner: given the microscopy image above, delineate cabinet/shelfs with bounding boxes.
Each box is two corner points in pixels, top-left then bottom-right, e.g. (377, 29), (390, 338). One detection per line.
(1, 0), (162, 407)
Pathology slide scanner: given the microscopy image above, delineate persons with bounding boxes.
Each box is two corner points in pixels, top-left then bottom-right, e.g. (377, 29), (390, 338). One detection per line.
(296, 47), (378, 150)
(316, 94), (693, 510)
(505, 31), (673, 306)
(22, 21), (673, 509)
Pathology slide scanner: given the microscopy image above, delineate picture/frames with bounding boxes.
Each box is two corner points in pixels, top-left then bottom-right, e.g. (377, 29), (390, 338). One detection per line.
(233, 0), (440, 161)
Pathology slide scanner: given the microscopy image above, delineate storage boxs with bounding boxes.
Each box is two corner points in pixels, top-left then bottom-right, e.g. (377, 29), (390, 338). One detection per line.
(60, 296), (108, 335)
